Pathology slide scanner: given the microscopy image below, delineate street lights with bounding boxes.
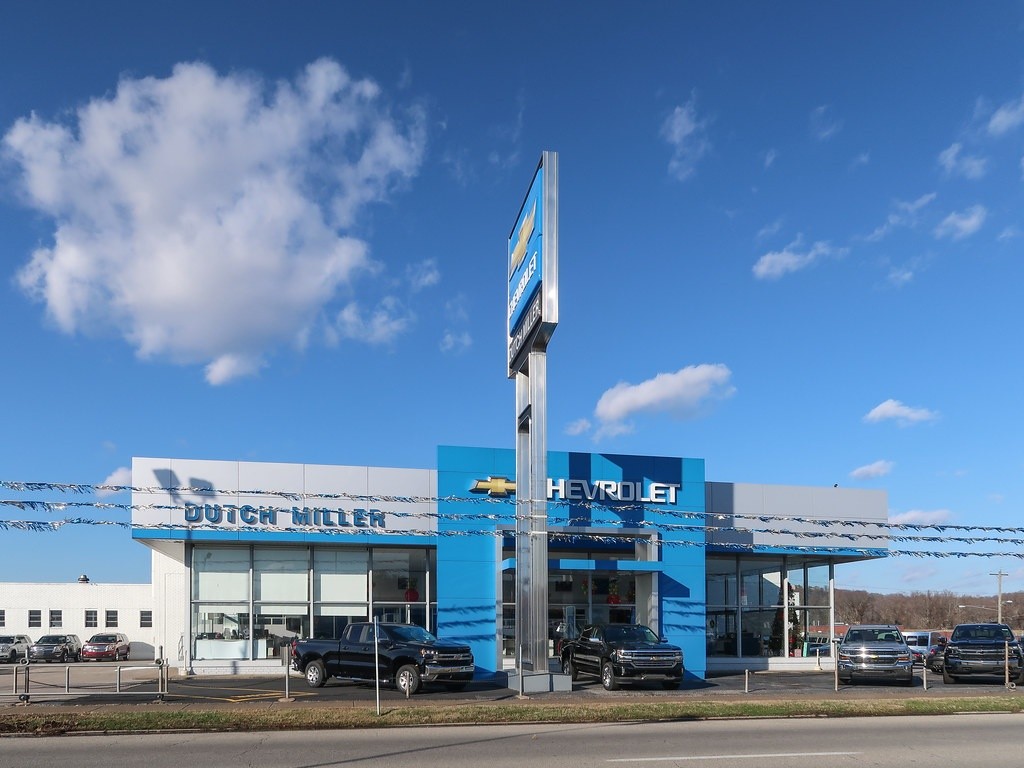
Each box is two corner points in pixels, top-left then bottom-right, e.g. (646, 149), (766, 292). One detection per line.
(958, 600), (1013, 624)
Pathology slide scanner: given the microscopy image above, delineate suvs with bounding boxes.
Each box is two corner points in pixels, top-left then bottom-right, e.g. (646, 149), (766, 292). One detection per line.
(0, 634), (33, 663)
(82, 633), (131, 662)
(926, 645), (945, 673)
(837, 624), (913, 685)
(943, 623), (1024, 685)
(28, 634), (82, 663)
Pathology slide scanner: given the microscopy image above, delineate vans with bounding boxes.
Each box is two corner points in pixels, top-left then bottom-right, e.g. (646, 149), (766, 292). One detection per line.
(893, 632), (941, 653)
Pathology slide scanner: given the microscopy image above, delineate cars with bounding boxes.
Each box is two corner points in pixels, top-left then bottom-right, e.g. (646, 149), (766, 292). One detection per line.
(810, 643), (830, 654)
(909, 649), (923, 665)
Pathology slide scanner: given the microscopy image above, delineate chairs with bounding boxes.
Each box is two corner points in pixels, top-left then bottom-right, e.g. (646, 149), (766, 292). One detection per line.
(848, 634), (863, 642)
(885, 634), (895, 640)
(994, 630), (1005, 639)
(962, 631), (972, 638)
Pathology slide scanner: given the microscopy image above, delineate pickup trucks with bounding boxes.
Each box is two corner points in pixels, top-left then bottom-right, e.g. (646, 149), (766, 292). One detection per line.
(292, 622), (475, 694)
(558, 622), (684, 691)
